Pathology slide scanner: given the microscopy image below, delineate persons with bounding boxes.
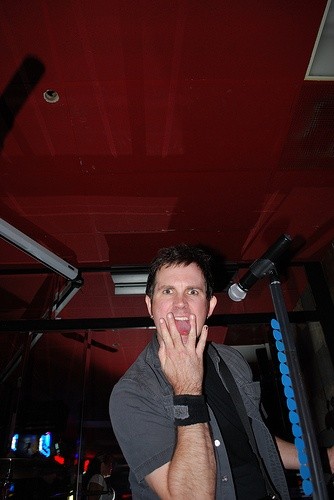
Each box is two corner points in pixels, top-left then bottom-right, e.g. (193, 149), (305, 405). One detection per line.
(23, 452), (116, 500)
(108, 248), (334, 500)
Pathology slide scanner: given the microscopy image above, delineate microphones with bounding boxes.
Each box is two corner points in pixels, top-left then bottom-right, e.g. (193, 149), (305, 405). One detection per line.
(228, 233), (291, 301)
